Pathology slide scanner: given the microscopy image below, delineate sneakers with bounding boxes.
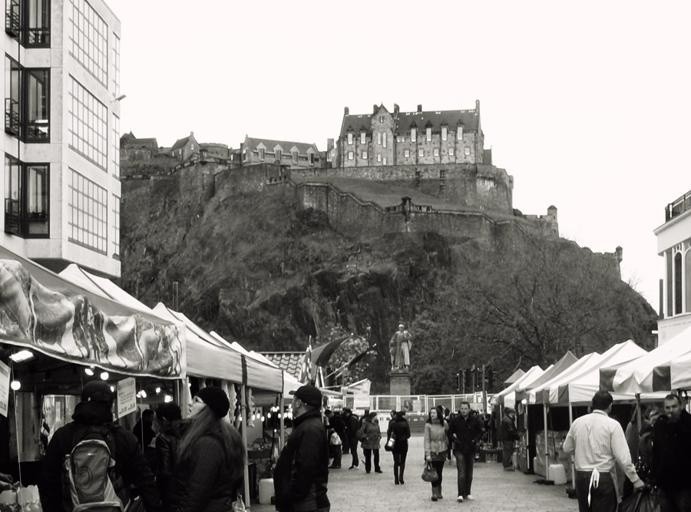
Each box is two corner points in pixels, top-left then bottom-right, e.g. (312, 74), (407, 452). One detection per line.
(457, 495), (473, 501)
(432, 494), (442, 501)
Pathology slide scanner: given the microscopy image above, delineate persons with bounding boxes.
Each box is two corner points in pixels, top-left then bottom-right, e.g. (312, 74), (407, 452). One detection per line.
(561, 390), (646, 512)
(152, 401), (184, 512)
(252, 406), (522, 474)
(390, 323), (414, 374)
(36, 380), (163, 512)
(423, 407), (457, 502)
(271, 384), (331, 511)
(131, 409), (156, 448)
(389, 411), (411, 484)
(650, 393), (690, 512)
(446, 401), (483, 504)
(164, 385), (246, 511)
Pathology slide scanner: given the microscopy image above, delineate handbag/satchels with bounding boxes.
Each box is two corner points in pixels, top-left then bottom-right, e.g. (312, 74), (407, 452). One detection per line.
(385, 437), (395, 451)
(422, 468), (438, 482)
(331, 432), (342, 445)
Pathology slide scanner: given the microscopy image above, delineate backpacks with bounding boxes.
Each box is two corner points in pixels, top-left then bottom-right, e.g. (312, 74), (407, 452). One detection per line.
(64, 433), (125, 512)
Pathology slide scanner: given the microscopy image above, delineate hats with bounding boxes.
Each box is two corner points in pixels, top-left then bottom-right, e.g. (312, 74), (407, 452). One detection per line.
(196, 387), (229, 417)
(289, 385), (321, 404)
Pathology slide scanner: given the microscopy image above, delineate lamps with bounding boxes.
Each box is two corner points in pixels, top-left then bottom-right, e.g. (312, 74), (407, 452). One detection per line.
(237, 404), (294, 423)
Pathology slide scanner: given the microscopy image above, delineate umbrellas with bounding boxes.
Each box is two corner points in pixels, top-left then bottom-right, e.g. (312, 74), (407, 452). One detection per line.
(297, 344), (312, 386)
